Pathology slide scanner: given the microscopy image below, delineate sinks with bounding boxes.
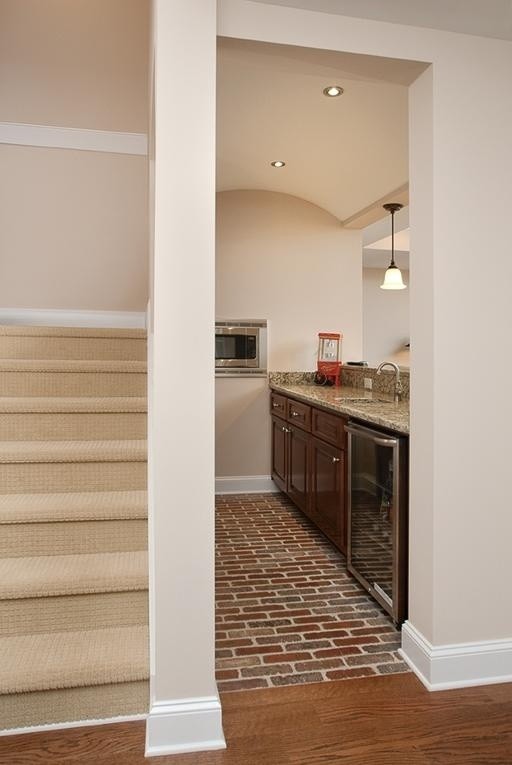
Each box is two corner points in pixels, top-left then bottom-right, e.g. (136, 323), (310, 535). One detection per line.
(337, 397), (391, 405)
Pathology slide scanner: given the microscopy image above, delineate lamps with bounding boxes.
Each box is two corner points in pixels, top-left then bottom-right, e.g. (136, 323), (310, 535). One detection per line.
(379, 203), (407, 291)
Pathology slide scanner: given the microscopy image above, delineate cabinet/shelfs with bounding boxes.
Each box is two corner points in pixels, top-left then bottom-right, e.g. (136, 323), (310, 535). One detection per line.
(269, 390), (312, 519)
(310, 407), (349, 555)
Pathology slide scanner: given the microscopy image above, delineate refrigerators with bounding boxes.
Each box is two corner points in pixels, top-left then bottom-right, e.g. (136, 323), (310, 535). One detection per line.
(345, 410), (409, 628)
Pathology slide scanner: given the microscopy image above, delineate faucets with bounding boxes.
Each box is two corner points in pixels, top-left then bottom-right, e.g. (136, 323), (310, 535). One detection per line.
(377, 362), (400, 400)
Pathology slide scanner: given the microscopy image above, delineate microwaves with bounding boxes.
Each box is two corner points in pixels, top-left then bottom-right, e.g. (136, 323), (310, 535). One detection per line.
(212, 324), (260, 368)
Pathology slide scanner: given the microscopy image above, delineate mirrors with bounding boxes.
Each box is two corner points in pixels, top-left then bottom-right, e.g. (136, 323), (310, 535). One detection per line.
(361, 204), (412, 371)
